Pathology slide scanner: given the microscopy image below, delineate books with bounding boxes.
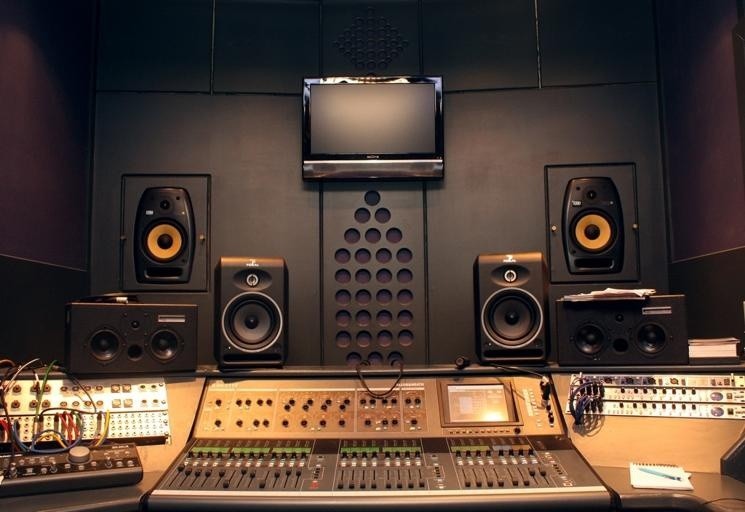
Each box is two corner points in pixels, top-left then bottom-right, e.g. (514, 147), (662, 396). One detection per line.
(629, 462), (694, 491)
(689, 344), (738, 358)
(689, 335), (742, 345)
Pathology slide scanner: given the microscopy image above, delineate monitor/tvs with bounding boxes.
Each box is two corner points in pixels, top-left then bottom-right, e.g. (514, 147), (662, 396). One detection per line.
(301, 77), (444, 183)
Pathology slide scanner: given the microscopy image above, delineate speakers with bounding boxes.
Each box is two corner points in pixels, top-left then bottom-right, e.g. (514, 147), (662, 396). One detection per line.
(556, 294), (689, 370)
(134, 188), (195, 284)
(474, 252), (551, 367)
(66, 300), (197, 375)
(563, 178), (623, 274)
(214, 257), (289, 370)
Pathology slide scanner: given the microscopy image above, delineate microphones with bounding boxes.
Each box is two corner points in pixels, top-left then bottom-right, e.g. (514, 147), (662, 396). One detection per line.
(455, 355), (552, 396)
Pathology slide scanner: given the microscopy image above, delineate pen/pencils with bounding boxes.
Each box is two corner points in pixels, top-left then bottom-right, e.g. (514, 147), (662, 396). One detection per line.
(640, 468), (682, 481)
